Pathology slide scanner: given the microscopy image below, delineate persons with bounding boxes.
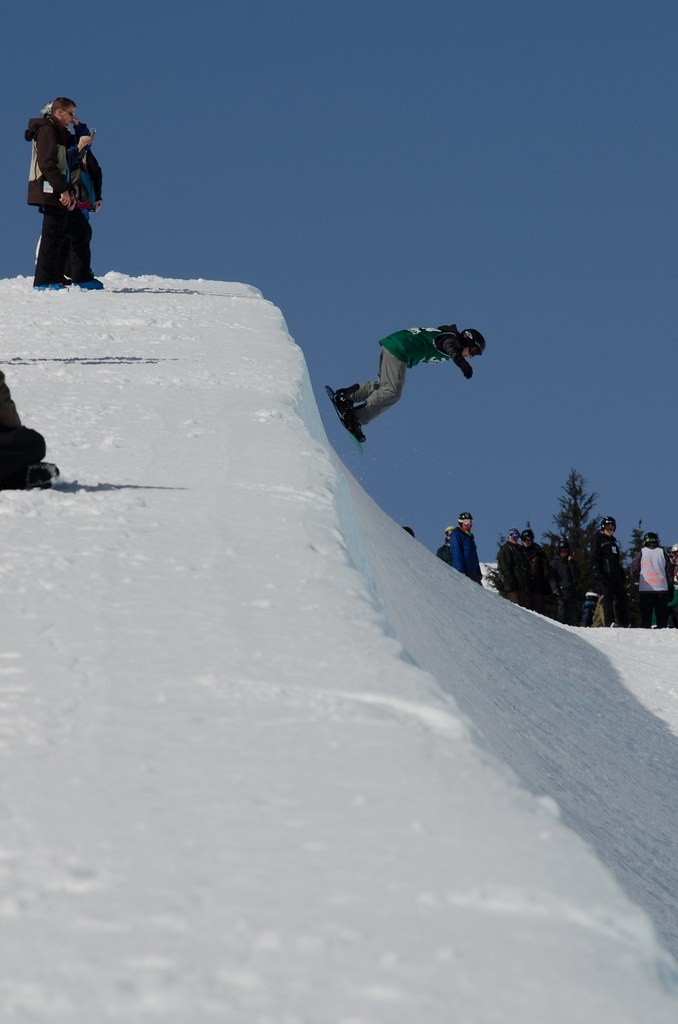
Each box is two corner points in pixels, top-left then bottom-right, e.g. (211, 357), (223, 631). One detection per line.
(436, 512), (482, 586)
(495, 516), (678, 630)
(0, 370), (59, 489)
(25, 97), (104, 290)
(325, 322), (486, 445)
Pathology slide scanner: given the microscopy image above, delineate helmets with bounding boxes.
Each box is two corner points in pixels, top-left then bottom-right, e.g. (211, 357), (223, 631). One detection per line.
(558, 541), (570, 550)
(643, 532), (659, 546)
(444, 526), (455, 533)
(461, 328), (486, 356)
(457, 512), (473, 525)
(672, 544), (678, 551)
(601, 516), (616, 529)
(520, 530), (534, 538)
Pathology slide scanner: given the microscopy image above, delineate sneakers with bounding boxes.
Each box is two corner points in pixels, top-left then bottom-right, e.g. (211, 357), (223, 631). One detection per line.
(333, 393), (353, 415)
(73, 278), (103, 290)
(34, 283), (69, 291)
(344, 413), (366, 442)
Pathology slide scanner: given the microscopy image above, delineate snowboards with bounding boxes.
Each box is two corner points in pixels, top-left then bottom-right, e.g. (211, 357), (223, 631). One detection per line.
(324, 385), (364, 453)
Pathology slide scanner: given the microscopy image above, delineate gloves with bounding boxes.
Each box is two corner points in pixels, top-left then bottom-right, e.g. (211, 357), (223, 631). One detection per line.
(592, 566), (600, 576)
(554, 591), (562, 597)
(464, 370), (473, 379)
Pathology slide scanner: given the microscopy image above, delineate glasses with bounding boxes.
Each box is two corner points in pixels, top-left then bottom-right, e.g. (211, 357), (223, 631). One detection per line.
(458, 519), (473, 525)
(57, 108), (73, 117)
(522, 537), (532, 542)
(510, 534), (519, 538)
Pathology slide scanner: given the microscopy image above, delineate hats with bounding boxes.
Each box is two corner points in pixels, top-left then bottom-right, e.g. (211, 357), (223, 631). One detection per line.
(508, 528), (520, 537)
